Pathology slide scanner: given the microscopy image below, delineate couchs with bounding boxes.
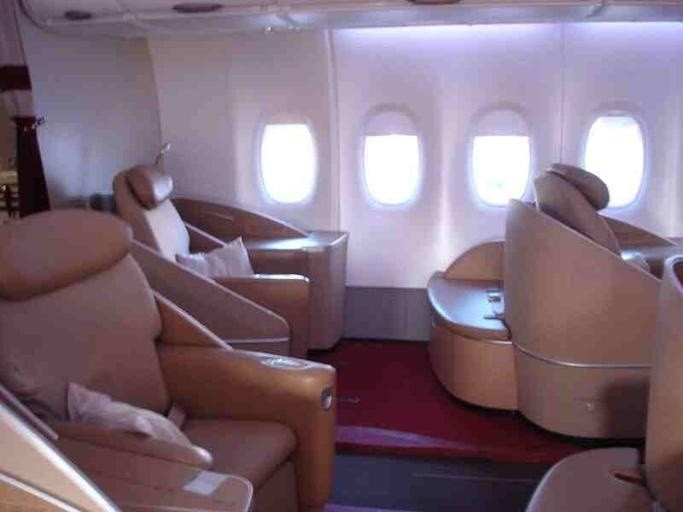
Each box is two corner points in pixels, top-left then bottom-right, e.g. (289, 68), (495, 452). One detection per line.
(0, 208), (339, 512)
(504, 164), (681, 441)
(112, 164), (349, 361)
(642, 254), (683, 510)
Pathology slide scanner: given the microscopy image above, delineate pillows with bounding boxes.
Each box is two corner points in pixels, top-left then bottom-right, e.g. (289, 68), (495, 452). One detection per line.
(172, 237), (254, 278)
(63, 376), (191, 449)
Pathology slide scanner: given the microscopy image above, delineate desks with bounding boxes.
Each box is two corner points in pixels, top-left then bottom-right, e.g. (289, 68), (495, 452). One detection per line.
(526, 444), (658, 512)
(423, 238), (519, 410)
